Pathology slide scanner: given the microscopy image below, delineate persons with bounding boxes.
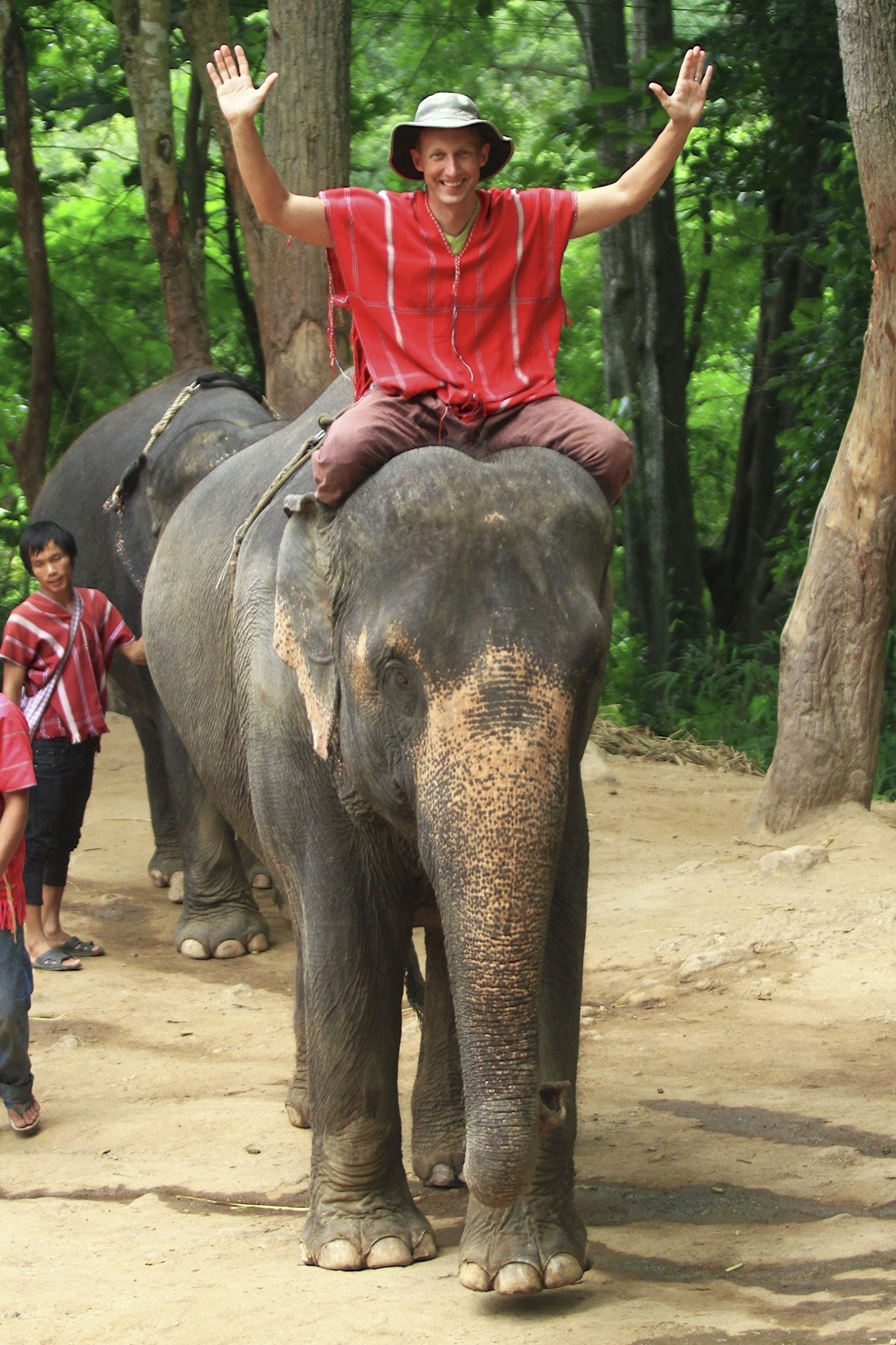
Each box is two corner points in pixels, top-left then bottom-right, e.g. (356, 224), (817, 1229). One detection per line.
(0, 521), (148, 971)
(206, 44), (713, 508)
(0, 693), (41, 1132)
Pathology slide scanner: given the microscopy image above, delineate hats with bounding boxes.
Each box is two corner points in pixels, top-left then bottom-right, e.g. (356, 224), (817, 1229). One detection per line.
(387, 93), (514, 183)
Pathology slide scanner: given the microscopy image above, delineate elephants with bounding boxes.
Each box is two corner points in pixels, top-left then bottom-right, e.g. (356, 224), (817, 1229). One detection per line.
(140, 364), (616, 1298)
(29, 364), (296, 961)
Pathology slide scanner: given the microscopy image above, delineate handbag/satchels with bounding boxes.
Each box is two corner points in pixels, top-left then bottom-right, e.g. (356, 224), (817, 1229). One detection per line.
(17, 690), (49, 743)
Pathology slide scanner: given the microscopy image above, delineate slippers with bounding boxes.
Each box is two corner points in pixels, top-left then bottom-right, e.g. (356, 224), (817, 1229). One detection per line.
(28, 947), (83, 972)
(57, 935), (106, 958)
(3, 1092), (42, 1133)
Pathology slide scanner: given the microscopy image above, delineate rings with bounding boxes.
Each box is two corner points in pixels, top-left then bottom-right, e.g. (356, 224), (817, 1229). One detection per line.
(694, 78), (701, 82)
